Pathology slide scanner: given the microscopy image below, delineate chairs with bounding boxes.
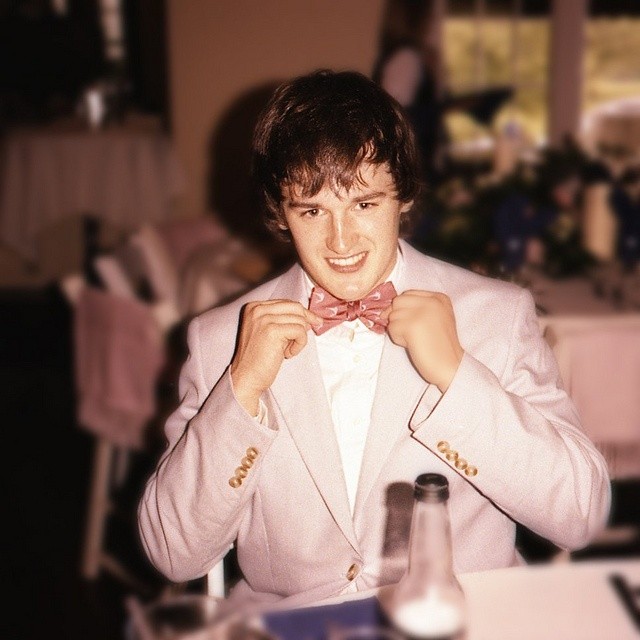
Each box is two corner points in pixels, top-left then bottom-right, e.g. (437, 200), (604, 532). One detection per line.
(66, 291), (228, 600)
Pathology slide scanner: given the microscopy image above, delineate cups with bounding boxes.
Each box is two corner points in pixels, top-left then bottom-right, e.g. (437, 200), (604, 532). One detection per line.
(126, 596), (273, 640)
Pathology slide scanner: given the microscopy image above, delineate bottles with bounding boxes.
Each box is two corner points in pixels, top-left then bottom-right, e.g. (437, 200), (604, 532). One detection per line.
(377, 473), (467, 638)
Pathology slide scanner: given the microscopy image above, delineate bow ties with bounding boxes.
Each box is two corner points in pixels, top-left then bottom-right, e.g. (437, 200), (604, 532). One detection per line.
(308, 281), (398, 336)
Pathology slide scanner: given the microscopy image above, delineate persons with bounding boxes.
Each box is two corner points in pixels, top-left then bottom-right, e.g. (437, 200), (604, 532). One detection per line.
(132, 64), (615, 622)
